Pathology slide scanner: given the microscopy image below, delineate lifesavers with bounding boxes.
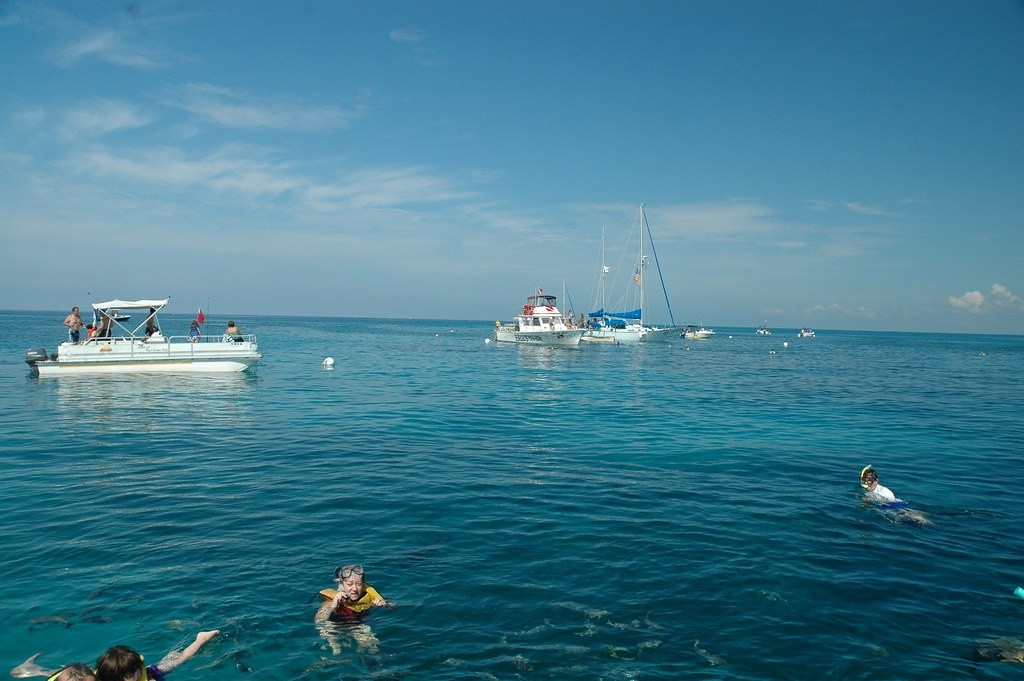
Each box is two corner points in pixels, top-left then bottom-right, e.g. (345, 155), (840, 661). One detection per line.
(523, 304), (533, 315)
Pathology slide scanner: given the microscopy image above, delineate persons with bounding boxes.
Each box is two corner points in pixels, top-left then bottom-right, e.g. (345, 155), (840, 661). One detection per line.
(63, 307), (86, 344)
(495, 312), (601, 331)
(80, 309), (114, 344)
(329, 564), (387, 608)
(145, 308), (155, 337)
(188, 322), (200, 343)
(862, 469), (895, 500)
(48, 630), (221, 681)
(226, 321), (244, 342)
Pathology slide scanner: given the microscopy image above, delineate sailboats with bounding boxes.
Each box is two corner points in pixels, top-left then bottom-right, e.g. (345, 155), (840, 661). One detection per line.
(563, 201), (683, 343)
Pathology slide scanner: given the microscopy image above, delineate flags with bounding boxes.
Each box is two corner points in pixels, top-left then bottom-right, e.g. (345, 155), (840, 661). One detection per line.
(198, 309), (204, 323)
(634, 267), (641, 284)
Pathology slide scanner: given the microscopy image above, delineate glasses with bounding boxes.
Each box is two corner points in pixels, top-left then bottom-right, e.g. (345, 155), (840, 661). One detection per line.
(861, 479), (875, 484)
(341, 565), (365, 579)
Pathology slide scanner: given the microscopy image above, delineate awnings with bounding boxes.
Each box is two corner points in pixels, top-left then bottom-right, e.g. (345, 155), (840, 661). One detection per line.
(91, 298), (168, 339)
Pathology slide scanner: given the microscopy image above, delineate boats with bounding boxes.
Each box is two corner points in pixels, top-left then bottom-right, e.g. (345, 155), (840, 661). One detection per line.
(27, 300), (263, 374)
(754, 322), (773, 336)
(678, 320), (715, 341)
(493, 287), (587, 346)
(797, 328), (817, 339)
